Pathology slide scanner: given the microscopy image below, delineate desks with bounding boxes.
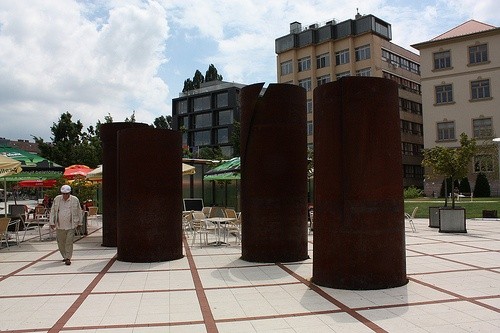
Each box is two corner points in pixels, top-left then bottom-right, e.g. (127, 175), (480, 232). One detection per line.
(25, 219), (50, 241)
(205, 217), (236, 246)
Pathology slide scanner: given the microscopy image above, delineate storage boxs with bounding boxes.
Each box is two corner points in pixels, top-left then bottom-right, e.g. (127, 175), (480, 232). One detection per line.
(483, 209), (497, 217)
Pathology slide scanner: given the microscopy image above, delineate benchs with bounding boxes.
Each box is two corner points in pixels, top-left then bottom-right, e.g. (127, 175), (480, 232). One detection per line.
(450, 191), (472, 201)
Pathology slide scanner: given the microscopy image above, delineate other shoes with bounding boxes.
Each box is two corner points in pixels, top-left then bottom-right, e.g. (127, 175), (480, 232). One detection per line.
(63, 259), (71, 265)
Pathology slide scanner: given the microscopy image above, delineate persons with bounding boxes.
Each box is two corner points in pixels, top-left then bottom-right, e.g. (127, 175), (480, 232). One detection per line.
(49, 185), (82, 265)
(454, 187), (459, 196)
(42, 194), (50, 208)
(81, 203), (89, 211)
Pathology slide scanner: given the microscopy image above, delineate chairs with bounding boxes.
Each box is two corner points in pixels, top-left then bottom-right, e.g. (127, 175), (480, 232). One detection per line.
(405, 206), (418, 232)
(308, 210), (313, 233)
(0, 207), (103, 251)
(182, 207), (242, 247)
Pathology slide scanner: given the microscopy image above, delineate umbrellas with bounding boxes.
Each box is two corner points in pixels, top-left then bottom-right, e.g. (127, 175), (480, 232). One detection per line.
(204, 157), (241, 180)
(0, 143), (65, 218)
(63, 165), (102, 185)
(182, 163), (195, 174)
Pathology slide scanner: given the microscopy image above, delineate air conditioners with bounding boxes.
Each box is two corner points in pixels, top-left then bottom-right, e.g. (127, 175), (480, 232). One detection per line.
(393, 63), (399, 69)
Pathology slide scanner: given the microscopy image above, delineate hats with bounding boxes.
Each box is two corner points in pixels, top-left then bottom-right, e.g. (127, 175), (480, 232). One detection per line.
(60, 185), (71, 193)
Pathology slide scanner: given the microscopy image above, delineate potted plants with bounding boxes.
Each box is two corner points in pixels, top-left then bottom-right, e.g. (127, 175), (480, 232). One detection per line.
(419, 132), (480, 233)
